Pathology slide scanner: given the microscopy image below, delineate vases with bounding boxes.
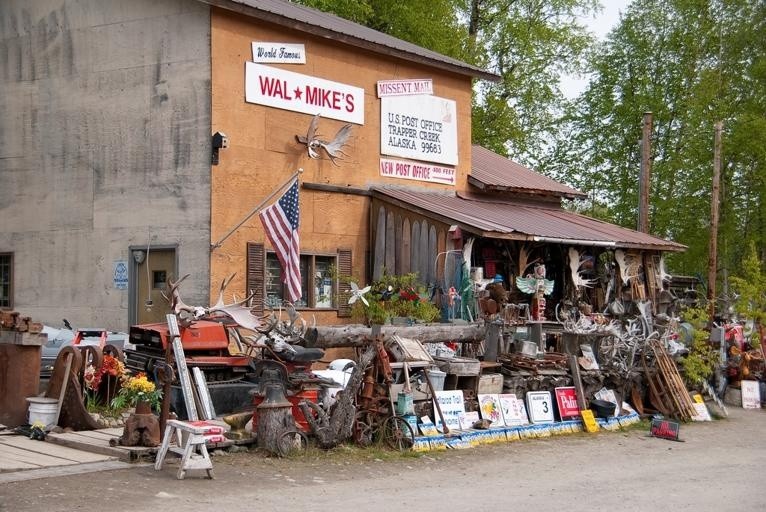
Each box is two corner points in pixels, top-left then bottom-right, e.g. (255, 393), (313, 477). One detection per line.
(135, 400), (152, 414)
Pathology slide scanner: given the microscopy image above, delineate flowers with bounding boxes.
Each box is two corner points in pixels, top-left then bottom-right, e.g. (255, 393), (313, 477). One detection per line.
(111, 372), (163, 413)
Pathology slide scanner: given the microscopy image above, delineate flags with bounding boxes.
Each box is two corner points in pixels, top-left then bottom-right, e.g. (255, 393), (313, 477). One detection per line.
(258, 177), (303, 305)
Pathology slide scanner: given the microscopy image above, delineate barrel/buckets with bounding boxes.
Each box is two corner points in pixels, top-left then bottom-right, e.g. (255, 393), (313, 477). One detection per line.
(29, 403), (58, 426)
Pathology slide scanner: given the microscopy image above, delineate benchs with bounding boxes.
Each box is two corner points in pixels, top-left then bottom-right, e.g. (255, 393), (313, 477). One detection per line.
(155, 418), (224, 479)
